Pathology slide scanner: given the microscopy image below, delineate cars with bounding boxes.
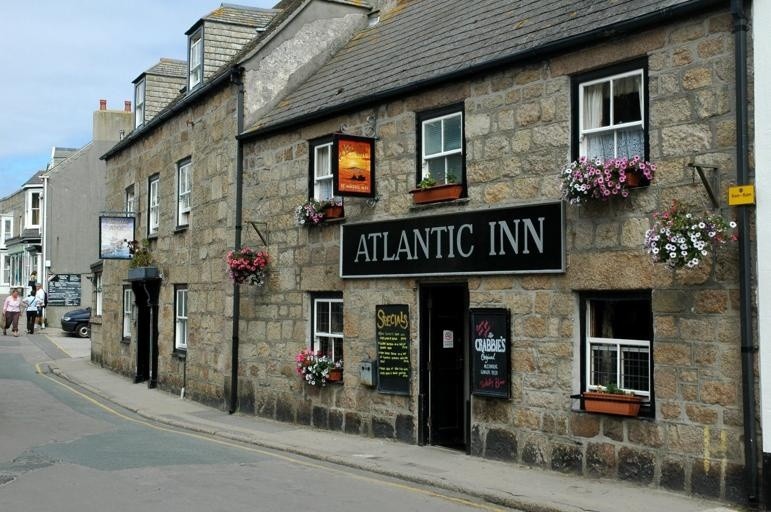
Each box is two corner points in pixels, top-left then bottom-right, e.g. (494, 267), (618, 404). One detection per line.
(62, 306), (92, 338)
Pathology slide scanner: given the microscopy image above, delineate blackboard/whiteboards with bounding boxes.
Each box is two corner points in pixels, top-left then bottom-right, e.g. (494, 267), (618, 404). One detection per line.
(468, 307), (512, 399)
(375, 304), (412, 397)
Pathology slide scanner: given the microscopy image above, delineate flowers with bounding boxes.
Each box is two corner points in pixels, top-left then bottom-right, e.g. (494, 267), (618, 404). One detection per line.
(296, 198), (343, 228)
(223, 246), (271, 289)
(644, 199), (739, 272)
(558, 154), (657, 209)
(295, 349), (344, 388)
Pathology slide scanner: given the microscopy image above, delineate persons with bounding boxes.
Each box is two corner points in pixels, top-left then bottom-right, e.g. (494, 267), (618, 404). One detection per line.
(35, 283), (46, 330)
(29, 270), (38, 296)
(2, 288), (23, 336)
(22, 289), (44, 334)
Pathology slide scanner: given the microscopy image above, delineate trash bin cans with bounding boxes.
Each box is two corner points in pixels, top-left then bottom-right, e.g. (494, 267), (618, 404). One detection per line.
(10, 286), (25, 316)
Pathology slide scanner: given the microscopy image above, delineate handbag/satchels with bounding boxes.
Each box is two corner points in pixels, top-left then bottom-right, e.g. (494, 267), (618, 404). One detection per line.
(0, 317), (6, 328)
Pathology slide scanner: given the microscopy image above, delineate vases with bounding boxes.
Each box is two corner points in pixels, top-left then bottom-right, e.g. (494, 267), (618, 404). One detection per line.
(617, 169), (640, 188)
(323, 206), (342, 219)
(325, 370), (342, 382)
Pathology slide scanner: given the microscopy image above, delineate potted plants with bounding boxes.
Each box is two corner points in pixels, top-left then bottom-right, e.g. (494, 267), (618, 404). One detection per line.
(124, 237), (158, 280)
(582, 382), (643, 417)
(408, 174), (462, 205)
